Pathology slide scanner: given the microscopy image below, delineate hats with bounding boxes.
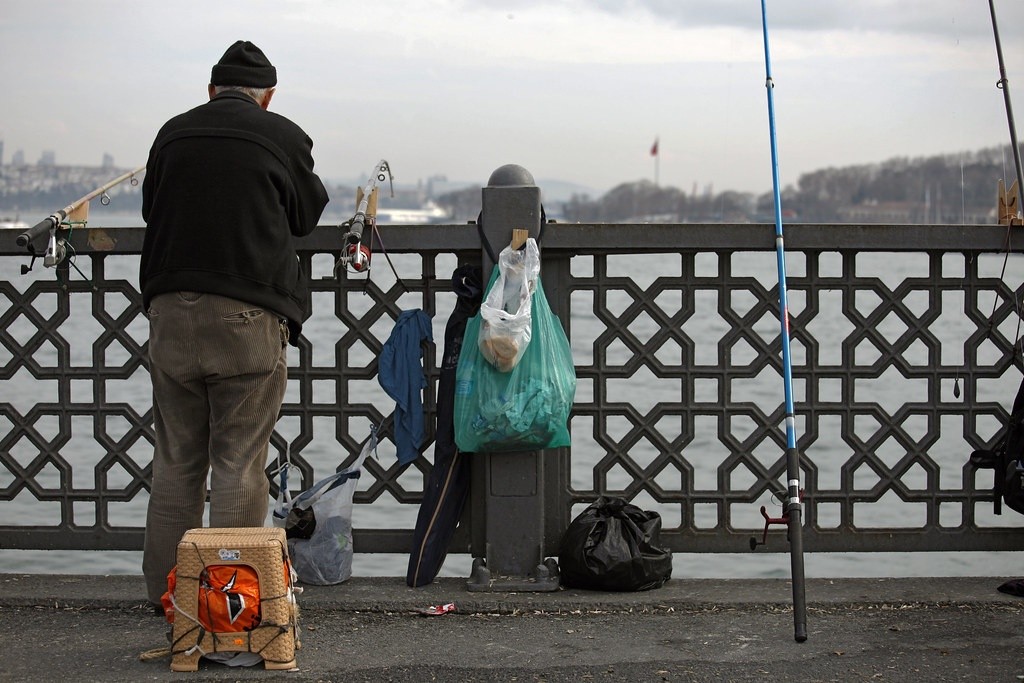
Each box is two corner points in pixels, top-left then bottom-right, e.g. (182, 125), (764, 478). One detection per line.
(210, 40), (276, 89)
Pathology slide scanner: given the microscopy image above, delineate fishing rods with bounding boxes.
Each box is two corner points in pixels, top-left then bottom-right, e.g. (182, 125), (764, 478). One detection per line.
(749, 1), (811, 642)
(322, 157), (395, 280)
(16, 163), (147, 276)
(987, 0), (1024, 231)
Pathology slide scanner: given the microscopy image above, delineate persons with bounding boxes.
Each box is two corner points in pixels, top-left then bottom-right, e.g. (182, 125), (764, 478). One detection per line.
(137, 38), (332, 621)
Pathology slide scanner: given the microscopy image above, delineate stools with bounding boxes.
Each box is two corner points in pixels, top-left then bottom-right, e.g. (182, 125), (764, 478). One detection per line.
(168, 526), (299, 671)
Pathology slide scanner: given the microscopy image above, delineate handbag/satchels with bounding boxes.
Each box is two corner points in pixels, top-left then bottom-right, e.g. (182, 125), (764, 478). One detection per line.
(271, 432), (380, 587)
(452, 236), (578, 452)
(558, 496), (673, 593)
